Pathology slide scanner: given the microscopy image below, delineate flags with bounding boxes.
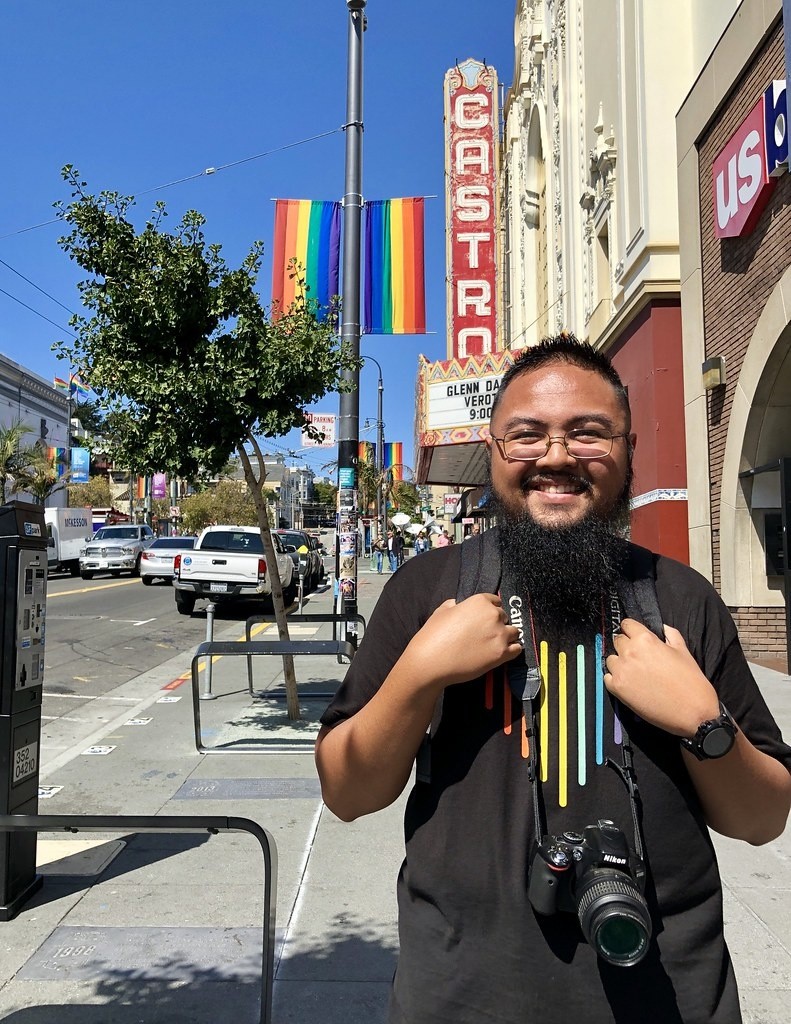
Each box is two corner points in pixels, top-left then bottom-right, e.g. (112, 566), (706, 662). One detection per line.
(54, 377), (70, 389)
(71, 374), (89, 398)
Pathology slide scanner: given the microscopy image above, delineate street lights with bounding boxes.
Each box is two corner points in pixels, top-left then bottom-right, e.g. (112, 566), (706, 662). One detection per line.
(292, 491), (305, 528)
(364, 417), (388, 542)
(134, 508), (146, 526)
(148, 512), (154, 527)
(65, 369), (90, 507)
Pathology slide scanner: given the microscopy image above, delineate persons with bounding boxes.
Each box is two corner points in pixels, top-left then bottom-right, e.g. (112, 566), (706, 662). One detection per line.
(392, 531), (406, 566)
(371, 534), (387, 575)
(312, 334), (790, 1024)
(465, 523), (480, 540)
(384, 531), (400, 572)
(415, 531), (429, 555)
(436, 530), (452, 547)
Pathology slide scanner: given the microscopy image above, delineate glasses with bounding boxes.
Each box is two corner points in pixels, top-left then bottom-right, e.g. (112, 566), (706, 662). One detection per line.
(490, 427), (628, 461)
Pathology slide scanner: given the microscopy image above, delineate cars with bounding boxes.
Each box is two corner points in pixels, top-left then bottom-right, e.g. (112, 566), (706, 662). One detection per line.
(307, 529), (320, 540)
(324, 519), (335, 527)
(140, 537), (201, 585)
(310, 537), (324, 584)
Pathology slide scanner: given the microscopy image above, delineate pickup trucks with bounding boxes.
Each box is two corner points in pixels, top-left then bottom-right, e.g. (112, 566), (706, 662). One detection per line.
(174, 526), (296, 615)
(268, 528), (321, 594)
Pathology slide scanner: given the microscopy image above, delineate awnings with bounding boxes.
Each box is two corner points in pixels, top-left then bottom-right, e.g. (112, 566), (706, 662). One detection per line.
(414, 345), (534, 487)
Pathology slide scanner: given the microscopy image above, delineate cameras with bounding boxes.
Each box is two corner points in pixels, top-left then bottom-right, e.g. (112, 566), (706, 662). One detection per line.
(529, 818), (653, 967)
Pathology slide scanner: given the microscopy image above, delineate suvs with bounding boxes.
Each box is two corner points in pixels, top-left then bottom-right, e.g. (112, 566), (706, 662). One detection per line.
(79, 524), (160, 580)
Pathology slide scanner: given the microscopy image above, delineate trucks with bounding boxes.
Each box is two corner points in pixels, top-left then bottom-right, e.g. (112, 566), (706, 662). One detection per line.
(44, 508), (94, 579)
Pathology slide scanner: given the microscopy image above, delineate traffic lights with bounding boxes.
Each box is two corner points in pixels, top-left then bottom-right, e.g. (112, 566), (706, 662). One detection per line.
(279, 518), (290, 529)
(429, 510), (434, 517)
(361, 506), (366, 515)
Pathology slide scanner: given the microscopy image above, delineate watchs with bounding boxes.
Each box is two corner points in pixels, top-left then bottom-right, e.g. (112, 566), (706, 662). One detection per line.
(682, 701), (739, 762)
